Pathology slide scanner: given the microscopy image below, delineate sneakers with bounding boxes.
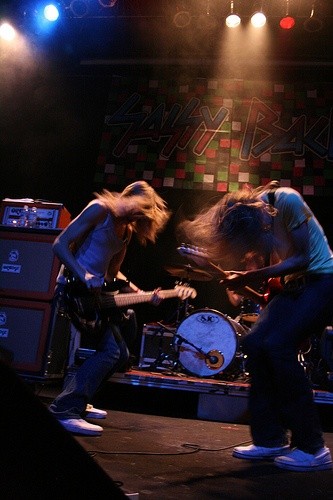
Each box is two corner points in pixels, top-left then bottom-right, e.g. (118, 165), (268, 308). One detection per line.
(56, 418), (103, 435)
(233, 444), (290, 460)
(273, 447), (333, 471)
(82, 404), (107, 418)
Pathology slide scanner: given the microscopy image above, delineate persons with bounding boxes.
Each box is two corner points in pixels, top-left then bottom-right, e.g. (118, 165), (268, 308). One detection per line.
(174, 179), (333, 473)
(50, 179), (172, 436)
(224, 250), (265, 372)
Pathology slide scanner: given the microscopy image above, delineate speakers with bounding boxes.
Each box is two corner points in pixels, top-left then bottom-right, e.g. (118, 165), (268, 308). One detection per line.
(0, 297), (69, 377)
(0, 232), (60, 300)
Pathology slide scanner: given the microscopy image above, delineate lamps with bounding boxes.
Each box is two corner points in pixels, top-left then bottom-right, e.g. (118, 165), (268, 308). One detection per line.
(225, 0), (297, 31)
